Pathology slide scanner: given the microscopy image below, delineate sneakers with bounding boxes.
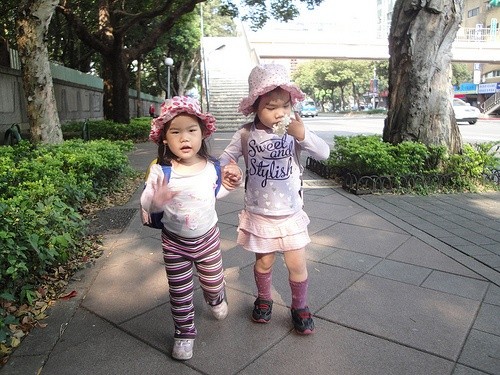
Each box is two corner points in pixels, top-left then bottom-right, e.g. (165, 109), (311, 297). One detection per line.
(252, 297), (273, 323)
(290, 303), (315, 335)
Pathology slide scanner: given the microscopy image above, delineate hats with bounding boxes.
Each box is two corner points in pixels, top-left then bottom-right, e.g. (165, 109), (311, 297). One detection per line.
(237, 63), (306, 117)
(149, 95), (216, 145)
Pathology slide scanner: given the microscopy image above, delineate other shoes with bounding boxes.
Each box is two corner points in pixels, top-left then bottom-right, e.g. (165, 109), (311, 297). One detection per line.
(211, 299), (229, 320)
(172, 339), (194, 360)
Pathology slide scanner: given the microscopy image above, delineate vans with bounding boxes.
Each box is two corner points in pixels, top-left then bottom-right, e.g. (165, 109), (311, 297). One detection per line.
(294, 99), (319, 118)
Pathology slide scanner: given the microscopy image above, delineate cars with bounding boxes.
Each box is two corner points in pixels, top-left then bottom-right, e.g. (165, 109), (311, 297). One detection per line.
(452, 97), (482, 125)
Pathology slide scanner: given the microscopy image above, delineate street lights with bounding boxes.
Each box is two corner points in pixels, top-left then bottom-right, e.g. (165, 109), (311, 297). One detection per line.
(165, 58), (173, 100)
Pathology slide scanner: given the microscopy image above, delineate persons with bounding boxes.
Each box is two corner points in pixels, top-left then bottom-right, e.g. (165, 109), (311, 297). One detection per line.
(149, 104), (155, 117)
(140, 97), (243, 359)
(220, 64), (330, 335)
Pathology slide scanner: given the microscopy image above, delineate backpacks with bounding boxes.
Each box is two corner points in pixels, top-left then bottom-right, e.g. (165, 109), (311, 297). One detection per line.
(141, 156), (222, 229)
